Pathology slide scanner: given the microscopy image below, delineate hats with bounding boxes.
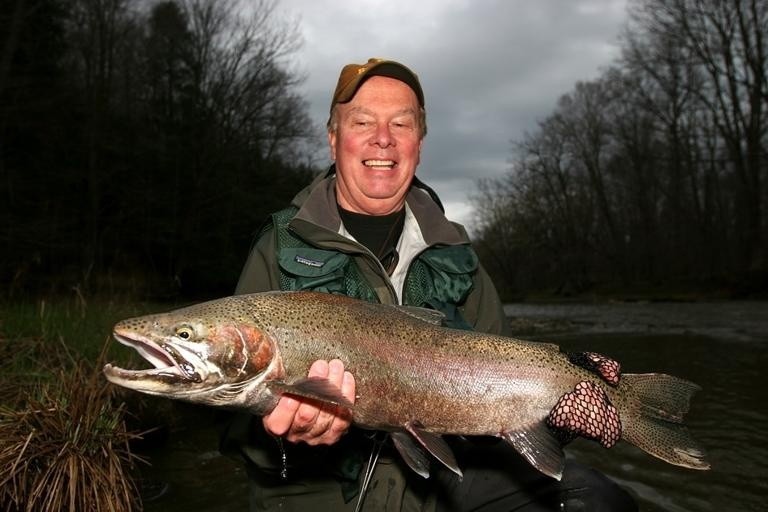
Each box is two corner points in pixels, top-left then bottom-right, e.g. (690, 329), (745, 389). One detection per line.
(327, 58), (425, 126)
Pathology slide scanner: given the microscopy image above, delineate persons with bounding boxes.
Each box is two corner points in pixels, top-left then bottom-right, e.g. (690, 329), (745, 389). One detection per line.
(220, 56), (641, 507)
